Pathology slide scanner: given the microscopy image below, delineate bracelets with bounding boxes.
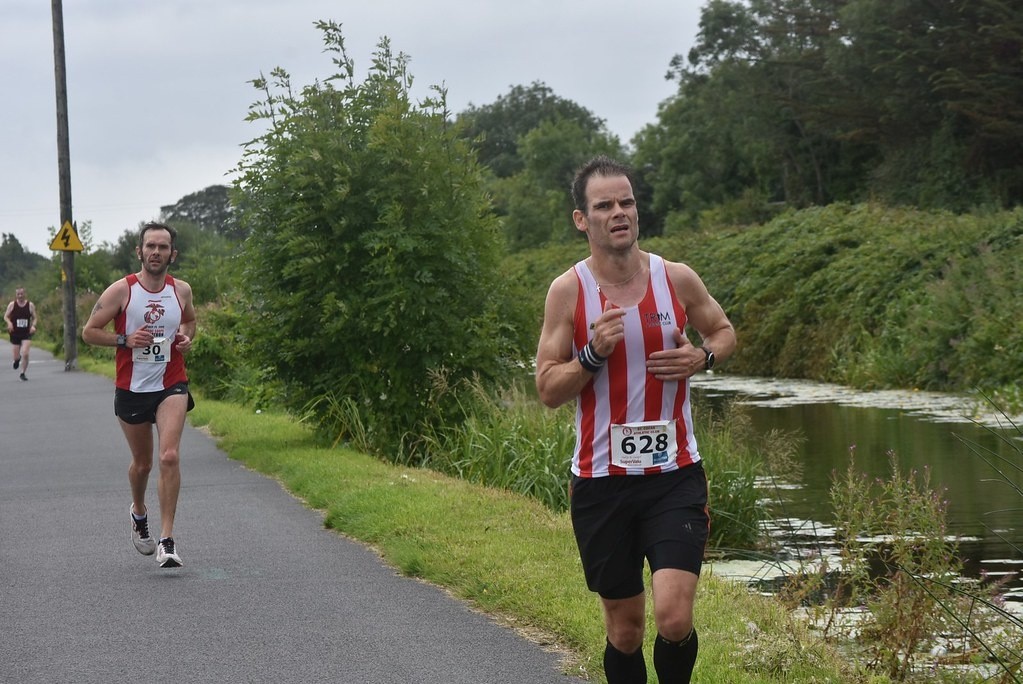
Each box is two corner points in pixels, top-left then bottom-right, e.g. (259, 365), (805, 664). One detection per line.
(578, 340), (606, 373)
(32, 324), (37, 329)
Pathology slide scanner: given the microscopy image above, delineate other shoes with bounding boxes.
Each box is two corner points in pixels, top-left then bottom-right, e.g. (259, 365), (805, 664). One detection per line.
(20, 373), (28, 381)
(14, 355), (21, 369)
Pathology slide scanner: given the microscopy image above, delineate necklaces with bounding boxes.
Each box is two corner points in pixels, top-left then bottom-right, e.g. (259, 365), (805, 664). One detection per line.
(590, 262), (641, 293)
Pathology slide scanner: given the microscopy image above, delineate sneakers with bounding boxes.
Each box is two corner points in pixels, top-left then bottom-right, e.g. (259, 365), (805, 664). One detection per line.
(130, 502), (155, 555)
(156, 539), (182, 568)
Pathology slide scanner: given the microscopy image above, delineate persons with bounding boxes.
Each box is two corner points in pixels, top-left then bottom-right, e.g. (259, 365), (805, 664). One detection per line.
(81, 221), (196, 568)
(4, 286), (38, 381)
(537, 154), (737, 684)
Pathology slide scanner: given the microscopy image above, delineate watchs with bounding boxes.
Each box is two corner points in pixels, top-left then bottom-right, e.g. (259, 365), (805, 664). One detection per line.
(700, 346), (715, 370)
(117, 334), (127, 348)
